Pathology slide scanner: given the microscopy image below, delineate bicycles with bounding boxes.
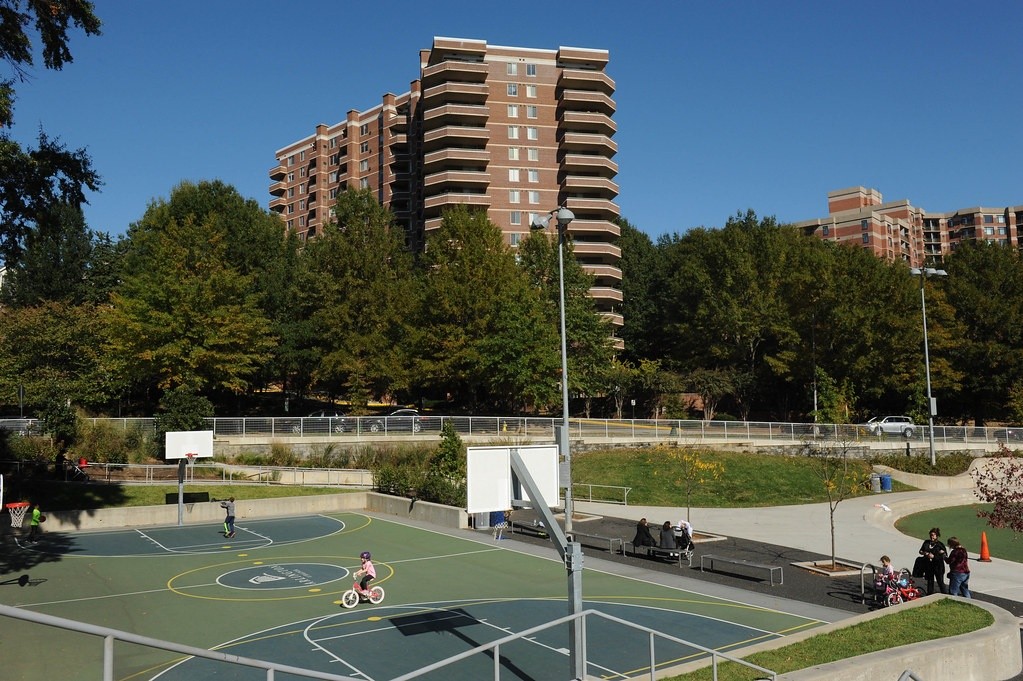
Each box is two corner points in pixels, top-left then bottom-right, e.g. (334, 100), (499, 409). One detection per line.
(342, 572), (385, 608)
(874, 568), (927, 607)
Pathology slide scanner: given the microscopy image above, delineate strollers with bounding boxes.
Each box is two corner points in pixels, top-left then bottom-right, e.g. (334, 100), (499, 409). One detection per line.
(65, 458), (93, 481)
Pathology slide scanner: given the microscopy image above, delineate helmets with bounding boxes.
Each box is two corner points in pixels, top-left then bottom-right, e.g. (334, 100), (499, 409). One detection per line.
(360, 551), (371, 559)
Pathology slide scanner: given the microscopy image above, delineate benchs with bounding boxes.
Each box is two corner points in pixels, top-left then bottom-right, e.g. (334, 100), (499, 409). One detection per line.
(650, 546), (693, 568)
(700, 553), (784, 586)
(623, 541), (656, 559)
(511, 519), (553, 542)
(566, 530), (623, 554)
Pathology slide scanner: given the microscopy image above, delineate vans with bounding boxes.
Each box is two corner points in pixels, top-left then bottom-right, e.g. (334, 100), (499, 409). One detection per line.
(865, 415), (916, 437)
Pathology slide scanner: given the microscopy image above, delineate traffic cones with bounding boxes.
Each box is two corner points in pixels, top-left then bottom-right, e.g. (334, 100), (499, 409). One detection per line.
(977, 531), (992, 563)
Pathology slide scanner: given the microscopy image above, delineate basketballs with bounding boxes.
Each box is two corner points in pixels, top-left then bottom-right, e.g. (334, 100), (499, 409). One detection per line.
(38, 516), (46, 522)
(221, 502), (226, 508)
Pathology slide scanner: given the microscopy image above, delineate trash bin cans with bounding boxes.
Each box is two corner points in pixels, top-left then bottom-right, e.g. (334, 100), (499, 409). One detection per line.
(490, 511), (505, 527)
(474, 512), (490, 528)
(869, 473), (881, 493)
(879, 473), (892, 491)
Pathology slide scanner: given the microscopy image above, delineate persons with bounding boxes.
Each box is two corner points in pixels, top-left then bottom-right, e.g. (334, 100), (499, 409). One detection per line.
(632, 517), (684, 560)
(491, 511), (509, 543)
(220, 497), (236, 538)
(354, 551), (377, 597)
(25, 502), (41, 544)
(877, 527), (972, 598)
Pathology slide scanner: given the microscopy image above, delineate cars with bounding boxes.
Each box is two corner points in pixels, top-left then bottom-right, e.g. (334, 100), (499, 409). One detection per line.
(185, 392), (304, 433)
(361, 407), (431, 433)
(925, 424), (966, 438)
(284, 408), (356, 434)
(994, 425), (1023, 440)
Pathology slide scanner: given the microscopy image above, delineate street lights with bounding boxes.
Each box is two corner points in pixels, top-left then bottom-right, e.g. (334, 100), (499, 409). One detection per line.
(907, 268), (949, 464)
(530, 207), (577, 540)
